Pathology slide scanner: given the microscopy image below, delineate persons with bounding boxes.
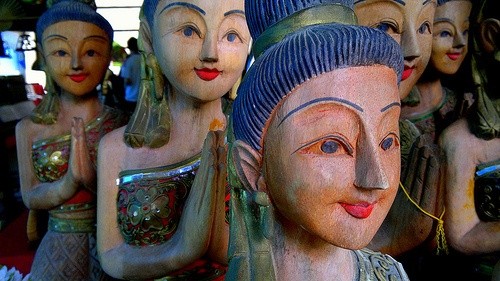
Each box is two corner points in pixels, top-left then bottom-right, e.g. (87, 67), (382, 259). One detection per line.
(13, 0), (124, 280)
(349, 0), (448, 281)
(225, 20), (412, 281)
(120, 37), (146, 116)
(436, 0), (499, 281)
(418, 0), (476, 148)
(96, 3), (251, 280)
(245, 0), (358, 60)
